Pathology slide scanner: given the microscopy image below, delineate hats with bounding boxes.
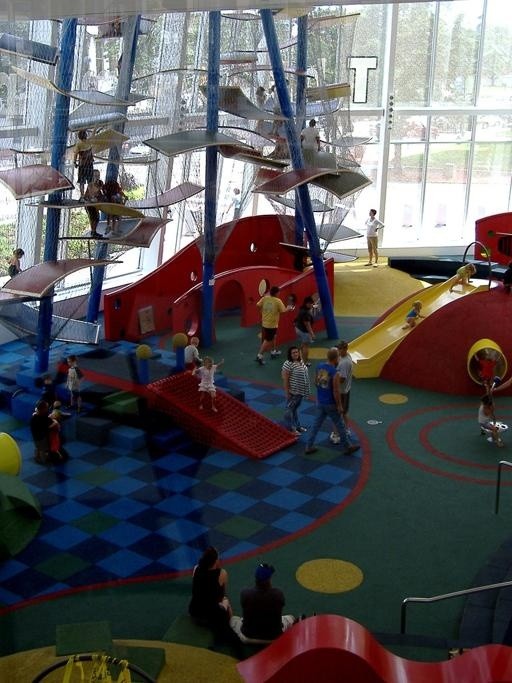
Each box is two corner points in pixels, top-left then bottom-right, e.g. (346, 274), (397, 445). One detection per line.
(254, 563), (274, 579)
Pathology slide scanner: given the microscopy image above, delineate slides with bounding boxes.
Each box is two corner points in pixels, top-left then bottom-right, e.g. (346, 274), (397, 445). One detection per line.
(347, 272), (499, 379)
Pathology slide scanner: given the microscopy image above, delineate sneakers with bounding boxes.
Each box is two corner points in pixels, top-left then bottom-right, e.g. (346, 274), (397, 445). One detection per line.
(329, 430), (360, 456)
(291, 426), (318, 453)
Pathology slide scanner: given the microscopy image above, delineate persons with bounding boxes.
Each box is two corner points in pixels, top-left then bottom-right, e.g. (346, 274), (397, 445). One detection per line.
(255, 78), (320, 152)
(73, 130), (128, 237)
(226, 189), (242, 219)
(401, 300), (426, 330)
(365, 209), (384, 268)
(30, 355), (85, 461)
(6, 249), (24, 278)
(478, 396), (505, 448)
(229, 562), (295, 643)
(255, 285), (295, 365)
(187, 546), (233, 630)
(280, 341), (361, 456)
(449, 264), (476, 292)
(191, 357), (225, 413)
(183, 336), (202, 367)
(293, 296), (316, 367)
(474, 352), (502, 394)
